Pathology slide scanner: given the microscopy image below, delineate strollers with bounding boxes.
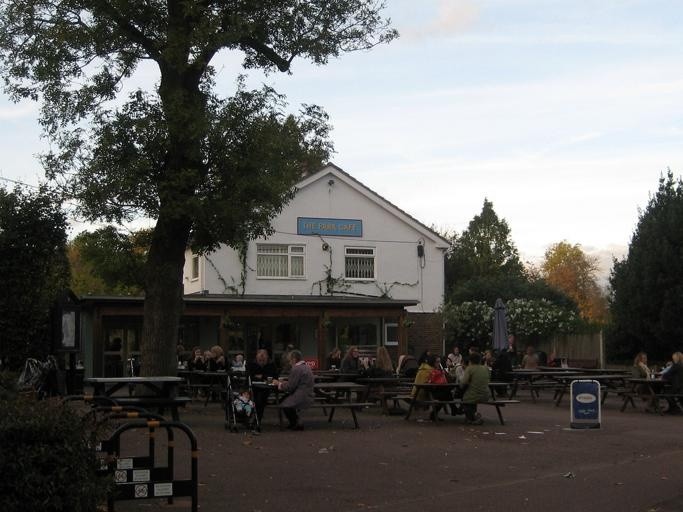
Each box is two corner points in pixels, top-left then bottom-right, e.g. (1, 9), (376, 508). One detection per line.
(225, 372), (261, 432)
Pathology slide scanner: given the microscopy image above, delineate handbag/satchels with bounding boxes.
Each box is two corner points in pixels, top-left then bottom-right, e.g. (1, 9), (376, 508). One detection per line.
(427, 369), (447, 384)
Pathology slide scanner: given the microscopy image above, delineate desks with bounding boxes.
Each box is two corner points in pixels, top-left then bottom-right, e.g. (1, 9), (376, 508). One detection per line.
(86, 376), (185, 423)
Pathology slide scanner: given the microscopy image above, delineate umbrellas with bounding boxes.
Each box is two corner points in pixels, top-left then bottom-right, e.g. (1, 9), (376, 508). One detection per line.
(493, 296), (509, 354)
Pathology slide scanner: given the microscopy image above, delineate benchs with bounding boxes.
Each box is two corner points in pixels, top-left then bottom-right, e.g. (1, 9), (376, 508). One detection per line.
(86, 396), (192, 407)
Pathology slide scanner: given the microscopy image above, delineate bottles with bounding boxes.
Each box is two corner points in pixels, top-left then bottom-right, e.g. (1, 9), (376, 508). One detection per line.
(278, 377), (285, 390)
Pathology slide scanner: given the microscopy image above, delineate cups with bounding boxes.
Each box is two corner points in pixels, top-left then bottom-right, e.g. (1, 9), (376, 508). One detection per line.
(267, 377), (273, 386)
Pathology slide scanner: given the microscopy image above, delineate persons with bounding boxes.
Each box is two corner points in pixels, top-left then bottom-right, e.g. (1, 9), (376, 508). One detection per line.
(660, 352), (683, 415)
(244, 349), (279, 425)
(323, 348), (342, 403)
(362, 348), (393, 409)
(340, 346), (371, 409)
(632, 353), (658, 413)
(233, 390), (256, 417)
(521, 346), (542, 403)
(410, 346), (524, 427)
(273, 350), (314, 431)
(174, 344), (255, 403)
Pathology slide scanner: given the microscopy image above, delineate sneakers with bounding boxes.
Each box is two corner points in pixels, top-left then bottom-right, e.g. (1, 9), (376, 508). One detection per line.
(496, 393), (509, 398)
(468, 413), (483, 425)
(430, 416), (445, 421)
(451, 408), (465, 416)
(285, 423), (305, 431)
